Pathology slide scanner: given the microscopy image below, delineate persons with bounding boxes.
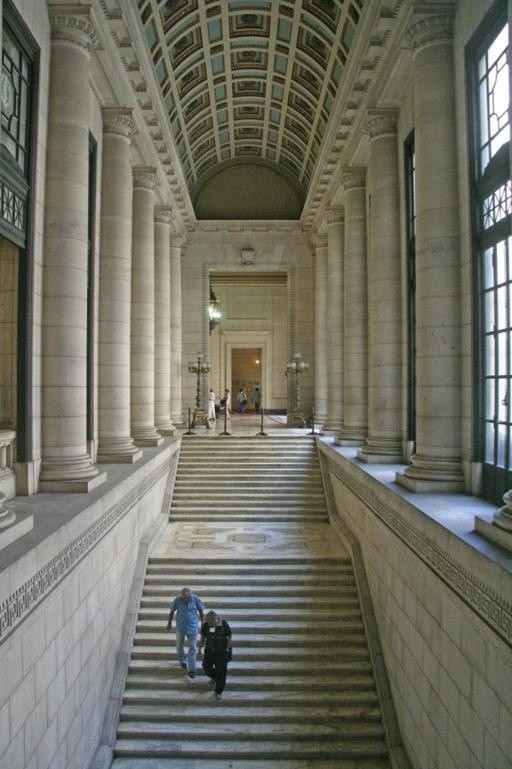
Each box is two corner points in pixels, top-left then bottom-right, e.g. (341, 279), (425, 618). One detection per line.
(235, 388), (247, 413)
(208, 388), (217, 420)
(221, 389), (230, 420)
(166, 588), (206, 679)
(250, 387), (262, 414)
(195, 610), (233, 701)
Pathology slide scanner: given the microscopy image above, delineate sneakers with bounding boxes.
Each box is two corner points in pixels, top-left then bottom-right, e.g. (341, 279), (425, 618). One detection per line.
(180, 661), (222, 701)
